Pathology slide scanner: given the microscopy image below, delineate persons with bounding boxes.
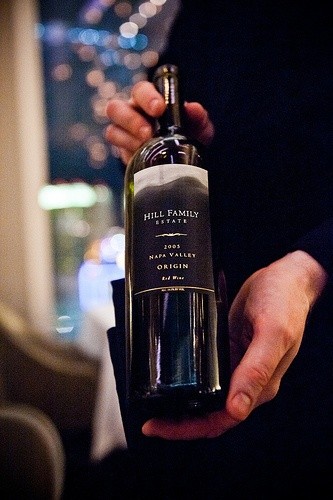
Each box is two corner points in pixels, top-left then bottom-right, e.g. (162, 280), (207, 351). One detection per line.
(105, 0), (333, 500)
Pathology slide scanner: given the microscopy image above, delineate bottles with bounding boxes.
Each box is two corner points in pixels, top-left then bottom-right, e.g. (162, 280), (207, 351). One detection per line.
(124, 66), (230, 424)
